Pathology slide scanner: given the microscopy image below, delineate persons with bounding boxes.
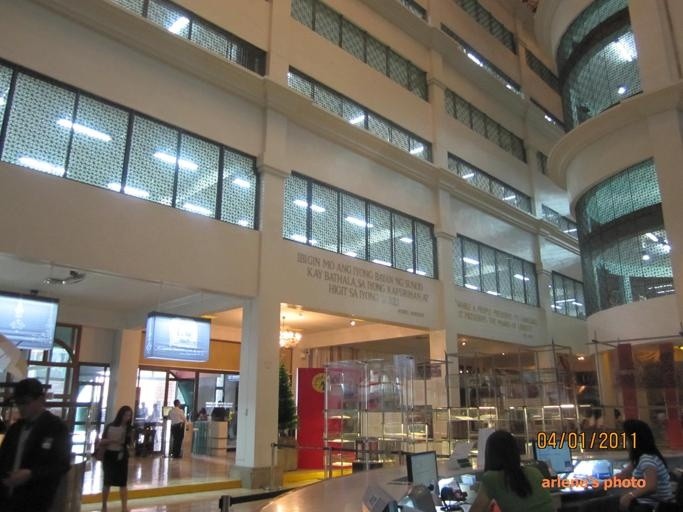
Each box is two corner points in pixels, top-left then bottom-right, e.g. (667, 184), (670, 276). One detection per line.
(100, 405), (134, 512)
(169, 400), (187, 459)
(600, 418), (674, 512)
(133, 399), (238, 447)
(467, 429), (557, 512)
(0, 376), (75, 511)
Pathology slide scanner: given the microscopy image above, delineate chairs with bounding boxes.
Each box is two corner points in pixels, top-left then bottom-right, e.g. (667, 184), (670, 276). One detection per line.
(630, 467), (683, 512)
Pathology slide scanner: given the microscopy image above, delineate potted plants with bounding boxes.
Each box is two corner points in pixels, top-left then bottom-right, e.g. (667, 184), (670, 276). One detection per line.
(274, 358), (299, 473)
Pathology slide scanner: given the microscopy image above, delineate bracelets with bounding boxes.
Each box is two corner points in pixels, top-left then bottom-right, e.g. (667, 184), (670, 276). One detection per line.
(627, 491), (636, 498)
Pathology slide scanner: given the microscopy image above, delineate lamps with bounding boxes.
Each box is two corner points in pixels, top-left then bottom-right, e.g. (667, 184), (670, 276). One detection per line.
(40, 268), (85, 288)
(641, 250), (649, 261)
(279, 303), (305, 351)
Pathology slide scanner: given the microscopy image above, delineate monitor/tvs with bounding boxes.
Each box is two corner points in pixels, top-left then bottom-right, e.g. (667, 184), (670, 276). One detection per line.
(406, 450), (439, 496)
(532, 439), (574, 474)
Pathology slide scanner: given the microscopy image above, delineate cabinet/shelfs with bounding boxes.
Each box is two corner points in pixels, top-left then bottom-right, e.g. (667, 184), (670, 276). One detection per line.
(323, 342), (582, 480)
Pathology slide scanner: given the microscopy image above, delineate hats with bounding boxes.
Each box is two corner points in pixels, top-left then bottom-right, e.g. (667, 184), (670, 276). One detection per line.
(7, 378), (51, 403)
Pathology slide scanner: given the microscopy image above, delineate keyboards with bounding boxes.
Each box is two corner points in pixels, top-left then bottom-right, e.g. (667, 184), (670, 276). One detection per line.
(560, 484), (593, 493)
